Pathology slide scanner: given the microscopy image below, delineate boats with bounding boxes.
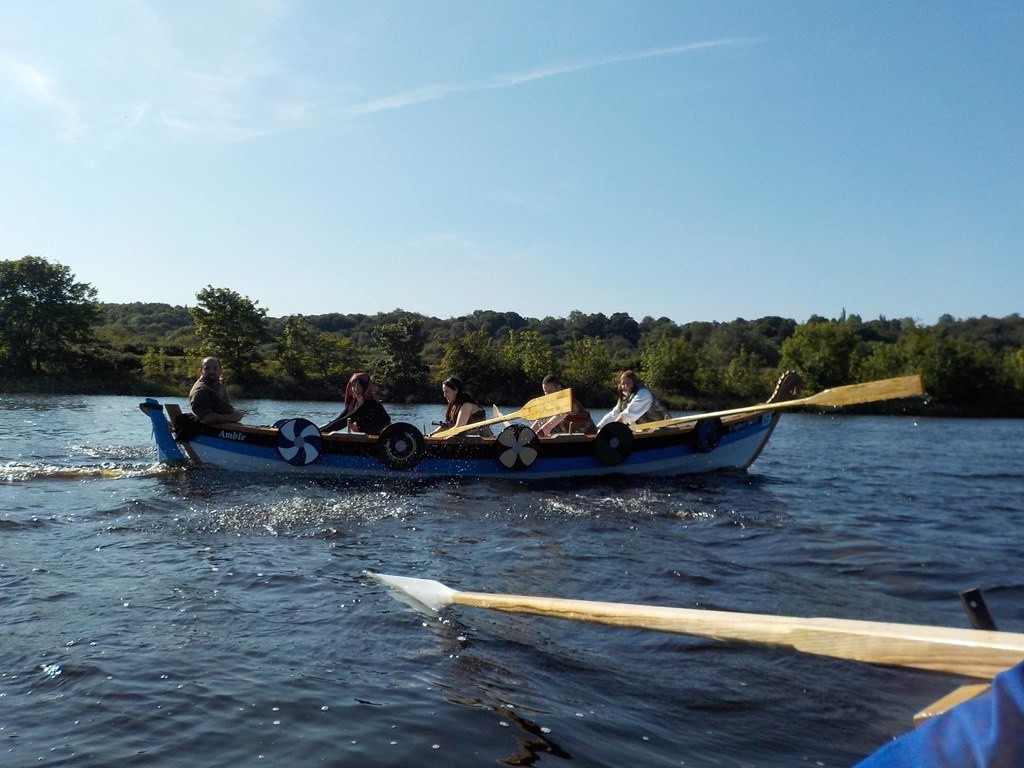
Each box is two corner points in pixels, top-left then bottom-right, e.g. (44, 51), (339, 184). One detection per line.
(138, 369), (801, 487)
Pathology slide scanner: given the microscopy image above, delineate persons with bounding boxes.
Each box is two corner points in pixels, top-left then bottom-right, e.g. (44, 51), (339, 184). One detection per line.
(531, 374), (598, 436)
(431, 376), (493, 437)
(595, 370), (672, 430)
(318, 373), (391, 436)
(189, 356), (244, 423)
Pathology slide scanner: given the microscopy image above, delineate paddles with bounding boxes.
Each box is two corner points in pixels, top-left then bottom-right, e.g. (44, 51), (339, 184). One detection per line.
(492, 402), (513, 428)
(428, 387), (574, 437)
(366, 569), (1024, 678)
(628, 374), (925, 431)
